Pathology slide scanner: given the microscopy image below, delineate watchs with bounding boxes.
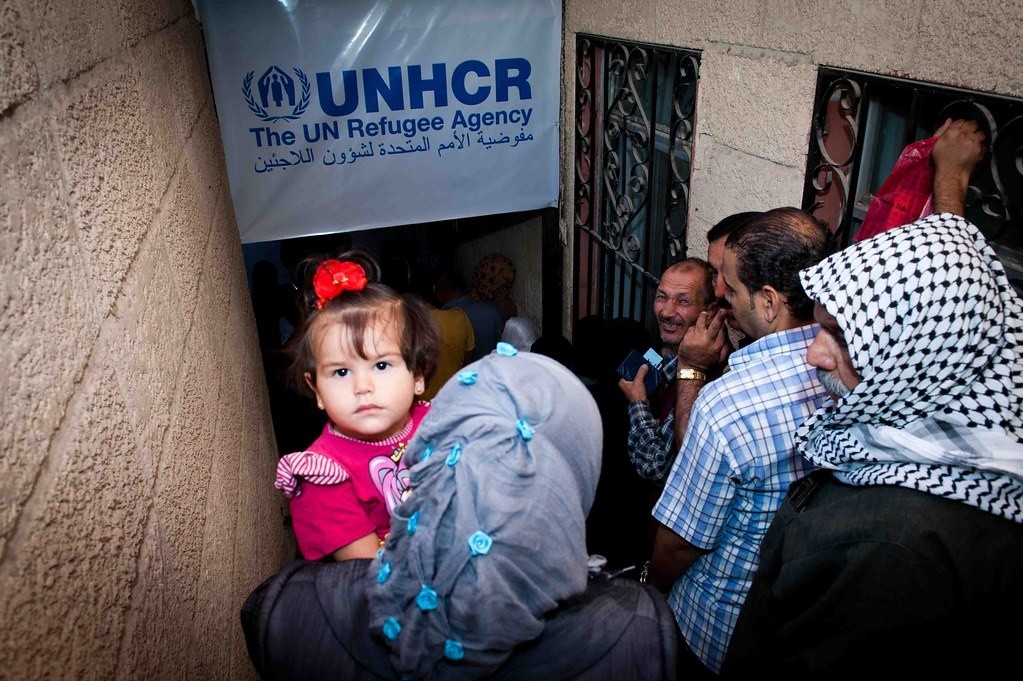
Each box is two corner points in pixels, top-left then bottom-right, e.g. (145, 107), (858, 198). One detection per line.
(675, 368), (709, 382)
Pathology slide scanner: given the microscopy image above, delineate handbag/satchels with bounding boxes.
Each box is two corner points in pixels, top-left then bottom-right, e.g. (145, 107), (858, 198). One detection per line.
(852, 136), (935, 242)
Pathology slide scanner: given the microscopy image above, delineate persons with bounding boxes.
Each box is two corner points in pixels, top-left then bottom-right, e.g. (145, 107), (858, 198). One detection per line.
(244, 203), (764, 587)
(638, 206), (841, 681)
(238, 341), (698, 681)
(273, 253), (442, 563)
(716, 118), (1023, 681)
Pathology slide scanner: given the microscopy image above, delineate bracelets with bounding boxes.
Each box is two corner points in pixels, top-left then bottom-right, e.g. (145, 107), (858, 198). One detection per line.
(640, 561), (651, 583)
